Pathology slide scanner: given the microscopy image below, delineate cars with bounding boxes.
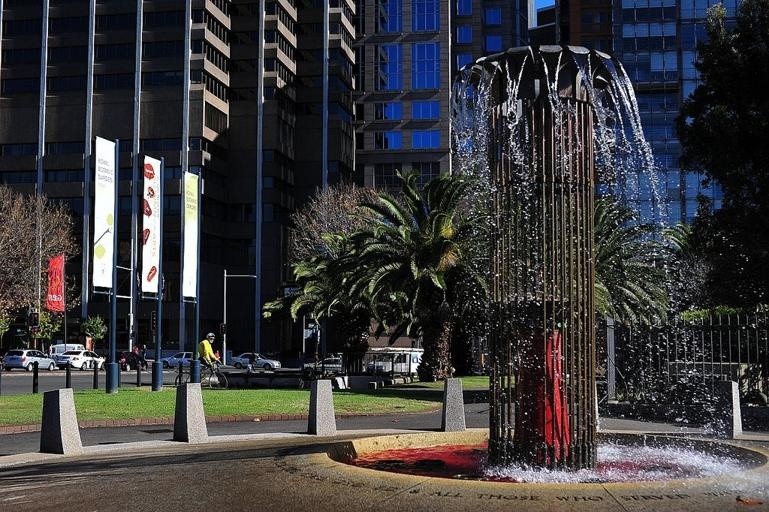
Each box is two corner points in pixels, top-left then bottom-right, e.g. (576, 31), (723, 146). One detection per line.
(231, 352), (282, 371)
(300, 356), (344, 379)
(55, 350), (107, 371)
(103, 351), (149, 371)
(2, 348), (58, 371)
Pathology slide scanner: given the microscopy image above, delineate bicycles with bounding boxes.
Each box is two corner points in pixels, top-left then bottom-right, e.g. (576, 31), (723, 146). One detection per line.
(174, 358), (231, 390)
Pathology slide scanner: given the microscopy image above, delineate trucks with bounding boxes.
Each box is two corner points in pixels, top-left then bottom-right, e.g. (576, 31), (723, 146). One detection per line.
(368, 347), (425, 373)
(44, 343), (86, 357)
(658, 361), (763, 400)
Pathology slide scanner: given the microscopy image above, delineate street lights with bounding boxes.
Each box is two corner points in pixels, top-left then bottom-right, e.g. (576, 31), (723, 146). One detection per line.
(221, 269), (260, 363)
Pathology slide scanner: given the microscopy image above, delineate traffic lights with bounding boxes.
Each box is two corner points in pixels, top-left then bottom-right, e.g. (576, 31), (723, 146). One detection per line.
(27, 312), (40, 328)
(220, 322), (229, 336)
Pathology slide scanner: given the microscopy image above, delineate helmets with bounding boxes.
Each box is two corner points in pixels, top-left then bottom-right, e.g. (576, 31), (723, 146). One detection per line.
(206, 333), (215, 338)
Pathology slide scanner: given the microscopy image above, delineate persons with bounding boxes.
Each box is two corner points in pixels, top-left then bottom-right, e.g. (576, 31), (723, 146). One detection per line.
(119, 344), (149, 371)
(48, 343), (53, 358)
(215, 349), (220, 367)
(198, 333), (221, 384)
(249, 351), (257, 372)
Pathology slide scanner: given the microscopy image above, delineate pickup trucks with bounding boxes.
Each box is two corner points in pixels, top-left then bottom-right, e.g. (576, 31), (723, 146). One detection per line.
(160, 351), (198, 370)
